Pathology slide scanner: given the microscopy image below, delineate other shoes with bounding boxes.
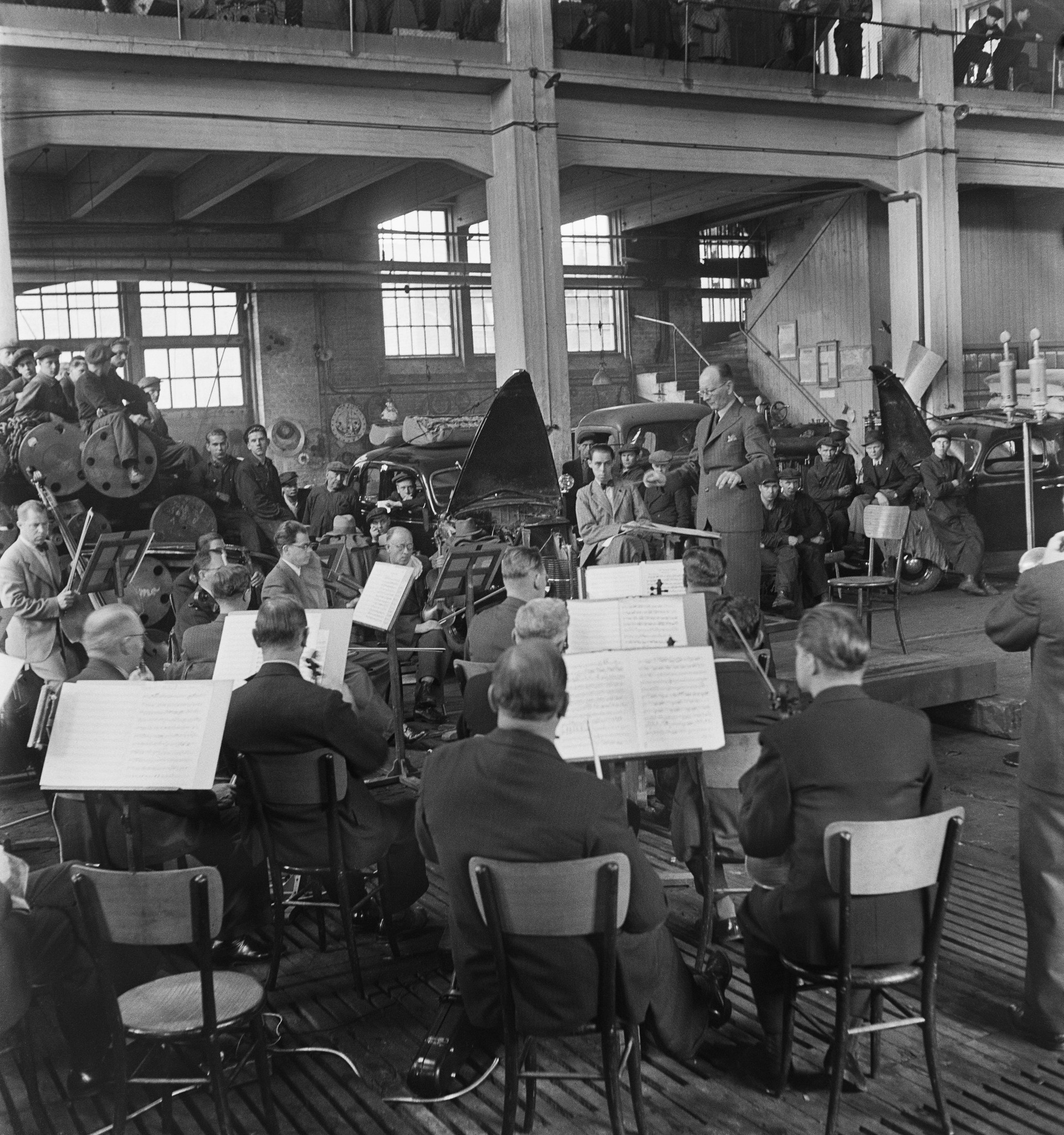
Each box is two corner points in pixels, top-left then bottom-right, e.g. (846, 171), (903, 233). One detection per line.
(1004, 1005), (1063, 1051)
(354, 903), (445, 939)
(67, 1066), (127, 1097)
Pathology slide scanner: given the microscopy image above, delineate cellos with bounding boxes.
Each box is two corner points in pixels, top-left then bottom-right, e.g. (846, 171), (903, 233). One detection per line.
(241, 547), (263, 612)
(31, 476), (169, 680)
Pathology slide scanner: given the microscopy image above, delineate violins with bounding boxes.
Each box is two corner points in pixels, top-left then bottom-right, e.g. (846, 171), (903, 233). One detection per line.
(420, 534), (456, 629)
(321, 566), (365, 601)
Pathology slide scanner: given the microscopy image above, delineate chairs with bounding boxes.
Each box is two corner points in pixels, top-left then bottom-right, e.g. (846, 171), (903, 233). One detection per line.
(689, 727), (780, 972)
(770, 807), (967, 1135)
(67, 865), (280, 1135)
(468, 852), (651, 1134)
(235, 744), (406, 1000)
(826, 504), (913, 654)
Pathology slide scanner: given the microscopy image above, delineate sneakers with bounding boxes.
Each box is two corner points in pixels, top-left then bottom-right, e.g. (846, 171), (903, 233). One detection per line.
(738, 1044), (869, 1095)
(771, 594), (801, 620)
(695, 913), (743, 943)
(958, 574), (1000, 594)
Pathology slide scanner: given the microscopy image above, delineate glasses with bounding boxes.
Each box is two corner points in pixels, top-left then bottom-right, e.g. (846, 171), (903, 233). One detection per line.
(697, 383), (726, 396)
(390, 543), (414, 550)
(292, 542), (312, 550)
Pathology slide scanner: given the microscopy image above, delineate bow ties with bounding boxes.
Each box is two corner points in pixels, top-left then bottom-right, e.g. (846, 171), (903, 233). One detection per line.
(36, 542), (49, 551)
(601, 479), (614, 490)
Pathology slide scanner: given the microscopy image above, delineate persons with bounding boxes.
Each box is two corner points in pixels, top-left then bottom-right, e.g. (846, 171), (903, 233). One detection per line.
(413, 637), (736, 1068)
(835, 430), (915, 568)
(804, 435), (857, 558)
(650, 593), (803, 947)
(217, 593), (434, 946)
(0, 839), (166, 1092)
(916, 430), (999, 597)
(1, 334), (830, 798)
(824, 0), (1045, 94)
(989, 530), (1062, 1053)
(814, 430), (855, 472)
(55, 602), (291, 968)
(738, 602), (942, 1095)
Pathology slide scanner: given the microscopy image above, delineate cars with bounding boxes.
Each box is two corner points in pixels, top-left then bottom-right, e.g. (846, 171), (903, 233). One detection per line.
(350, 394), (1063, 570)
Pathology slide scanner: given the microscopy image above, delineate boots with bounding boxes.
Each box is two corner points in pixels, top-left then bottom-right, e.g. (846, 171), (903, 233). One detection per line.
(126, 462), (146, 484)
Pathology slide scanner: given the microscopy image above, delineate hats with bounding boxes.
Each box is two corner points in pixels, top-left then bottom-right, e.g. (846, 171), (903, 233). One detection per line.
(577, 428), (674, 465)
(452, 517), (483, 536)
(759, 420), (951, 486)
(279, 460), (415, 536)
(0, 339), (162, 388)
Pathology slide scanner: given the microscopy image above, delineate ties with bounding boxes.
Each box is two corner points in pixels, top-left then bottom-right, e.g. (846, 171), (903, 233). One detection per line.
(710, 413), (719, 434)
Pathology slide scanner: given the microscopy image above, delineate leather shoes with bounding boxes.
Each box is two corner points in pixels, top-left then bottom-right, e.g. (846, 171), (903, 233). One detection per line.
(210, 936), (287, 964)
(388, 700), (449, 746)
(704, 949), (733, 1027)
